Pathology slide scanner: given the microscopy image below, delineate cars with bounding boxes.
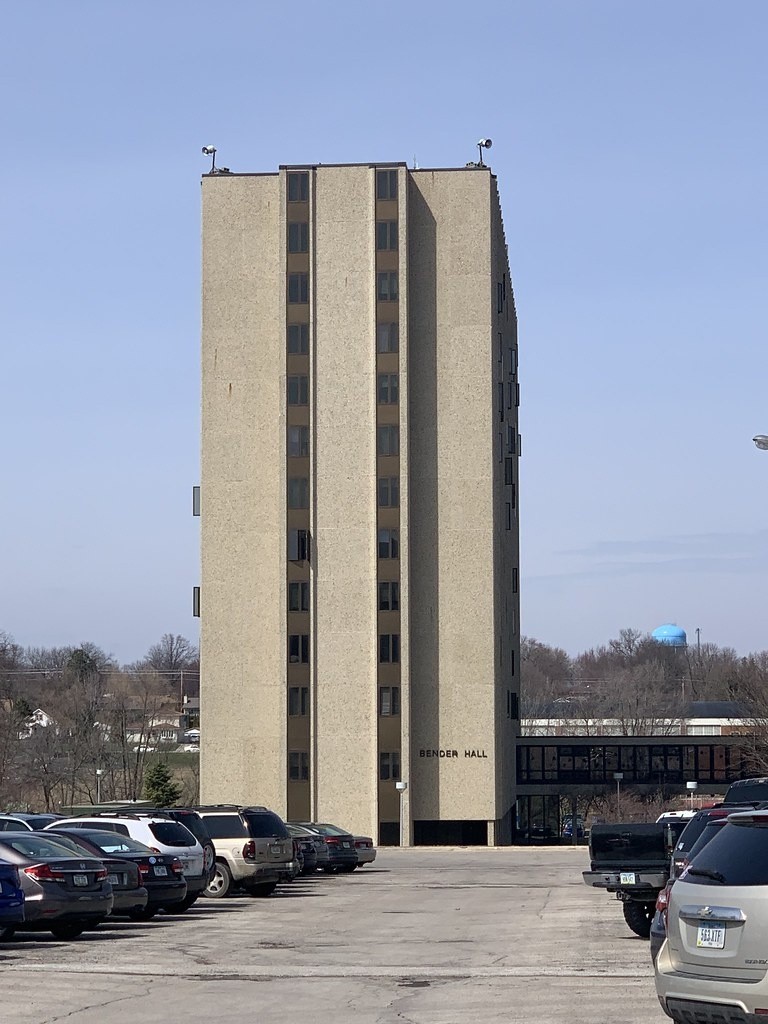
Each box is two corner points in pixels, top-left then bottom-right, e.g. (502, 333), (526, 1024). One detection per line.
(284, 823), (377, 877)
(132, 745), (158, 753)
(184, 745), (200, 753)
(562, 814), (582, 836)
(533, 819), (551, 836)
(36, 829), (188, 922)
(26, 832), (149, 930)
(0, 812), (68, 832)
(0, 830), (115, 942)
(0, 859), (25, 924)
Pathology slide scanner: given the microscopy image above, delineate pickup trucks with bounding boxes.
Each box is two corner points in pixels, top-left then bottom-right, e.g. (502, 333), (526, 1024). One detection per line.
(582, 777), (768, 940)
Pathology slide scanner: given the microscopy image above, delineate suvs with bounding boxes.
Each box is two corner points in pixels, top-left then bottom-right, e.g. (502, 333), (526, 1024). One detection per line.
(164, 805), (297, 898)
(649, 801), (768, 1024)
(43, 813), (208, 915)
(97, 807), (216, 914)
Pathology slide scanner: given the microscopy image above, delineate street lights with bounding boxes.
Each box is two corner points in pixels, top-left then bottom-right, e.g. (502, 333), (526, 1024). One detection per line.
(613, 773), (623, 815)
(96, 770), (104, 804)
(686, 781), (697, 809)
(396, 782), (407, 847)
